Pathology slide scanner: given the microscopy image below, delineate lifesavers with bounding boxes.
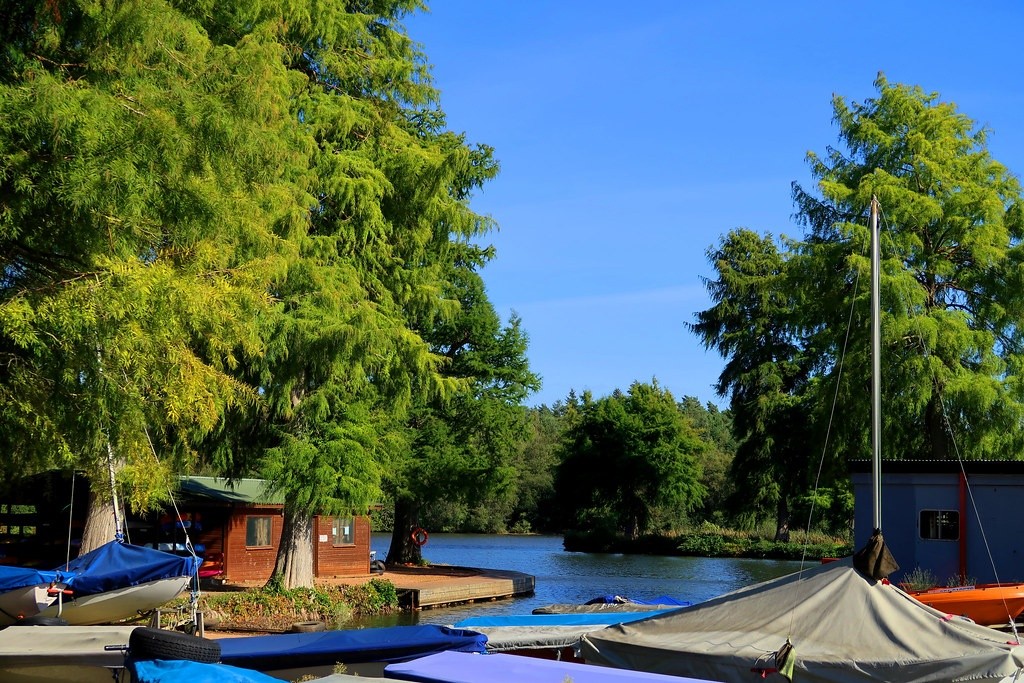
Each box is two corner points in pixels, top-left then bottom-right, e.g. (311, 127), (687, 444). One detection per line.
(412, 528), (428, 545)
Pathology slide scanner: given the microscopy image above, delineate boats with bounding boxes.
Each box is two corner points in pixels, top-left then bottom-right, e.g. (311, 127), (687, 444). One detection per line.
(0, 565), (77, 631)
(898, 581), (1024, 628)
(383, 648), (719, 683)
(21, 536), (205, 627)
(201, 624), (488, 681)
(103, 622), (292, 683)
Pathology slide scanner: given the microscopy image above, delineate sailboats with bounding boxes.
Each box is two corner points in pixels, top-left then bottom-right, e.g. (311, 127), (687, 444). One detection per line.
(559, 191), (1024, 682)
